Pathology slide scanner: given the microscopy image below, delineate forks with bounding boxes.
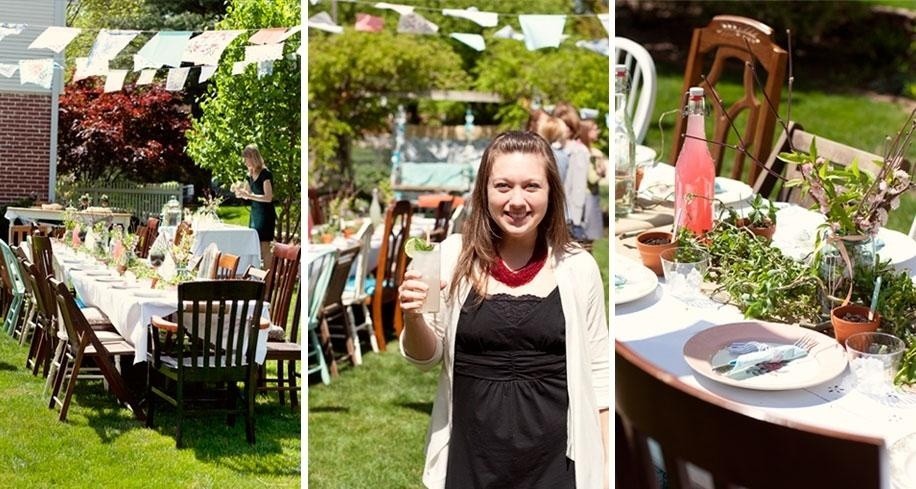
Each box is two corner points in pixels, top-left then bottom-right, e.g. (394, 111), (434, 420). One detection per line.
(710, 334), (824, 370)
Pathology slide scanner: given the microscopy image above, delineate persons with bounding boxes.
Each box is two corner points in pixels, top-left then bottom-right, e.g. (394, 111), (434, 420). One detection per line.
(518, 102), (607, 252)
(396, 132), (612, 489)
(233, 143), (275, 273)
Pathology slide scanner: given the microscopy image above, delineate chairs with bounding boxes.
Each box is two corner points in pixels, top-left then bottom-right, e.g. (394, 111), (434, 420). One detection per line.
(308, 184), (468, 388)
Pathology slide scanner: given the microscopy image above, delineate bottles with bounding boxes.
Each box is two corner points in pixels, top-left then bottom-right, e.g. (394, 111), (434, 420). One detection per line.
(167, 196), (180, 226)
(370, 189), (381, 226)
(674, 87), (714, 240)
(615, 65), (637, 215)
(307, 198), (313, 242)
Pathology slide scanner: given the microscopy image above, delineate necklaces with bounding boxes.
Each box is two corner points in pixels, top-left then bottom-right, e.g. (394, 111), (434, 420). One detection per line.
(478, 225), (549, 288)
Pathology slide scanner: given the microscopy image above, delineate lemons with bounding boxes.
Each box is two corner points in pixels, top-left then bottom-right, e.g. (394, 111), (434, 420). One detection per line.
(404, 236), (416, 257)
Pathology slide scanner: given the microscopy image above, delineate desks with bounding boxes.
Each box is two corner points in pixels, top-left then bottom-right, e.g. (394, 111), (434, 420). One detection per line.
(3, 207), (132, 234)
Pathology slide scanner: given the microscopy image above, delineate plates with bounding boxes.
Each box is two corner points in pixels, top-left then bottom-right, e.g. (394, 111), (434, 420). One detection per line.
(55, 246), (163, 297)
(613, 256), (658, 306)
(641, 175), (754, 203)
(684, 319), (848, 391)
(609, 143), (656, 163)
(873, 226), (916, 264)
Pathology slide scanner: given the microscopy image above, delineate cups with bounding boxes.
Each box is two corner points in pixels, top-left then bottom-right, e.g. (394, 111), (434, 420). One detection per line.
(660, 247), (709, 299)
(410, 243), (440, 314)
(846, 332), (905, 394)
